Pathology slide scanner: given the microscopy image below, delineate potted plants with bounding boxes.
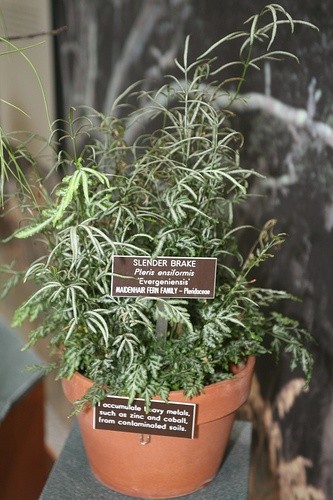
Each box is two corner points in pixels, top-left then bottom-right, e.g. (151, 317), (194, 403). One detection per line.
(0, 1), (328, 499)
